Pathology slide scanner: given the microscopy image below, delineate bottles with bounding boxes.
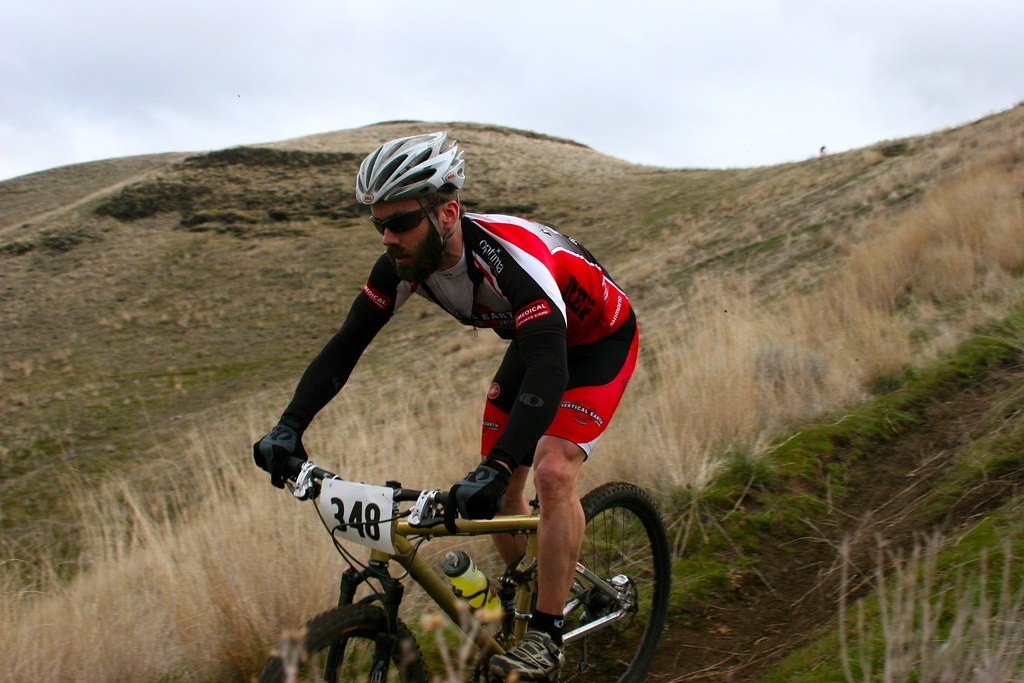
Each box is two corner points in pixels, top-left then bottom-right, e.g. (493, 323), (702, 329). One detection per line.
(441, 549), (503, 623)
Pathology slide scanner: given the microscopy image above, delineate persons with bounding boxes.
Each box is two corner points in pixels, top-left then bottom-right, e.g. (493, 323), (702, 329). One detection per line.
(253, 131), (640, 683)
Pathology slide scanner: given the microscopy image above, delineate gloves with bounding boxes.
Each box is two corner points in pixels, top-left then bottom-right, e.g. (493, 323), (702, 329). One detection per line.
(436, 459), (504, 533)
(253, 425), (308, 489)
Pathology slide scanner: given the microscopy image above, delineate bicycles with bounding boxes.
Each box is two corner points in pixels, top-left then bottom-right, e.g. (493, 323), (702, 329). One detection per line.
(256, 444), (673, 683)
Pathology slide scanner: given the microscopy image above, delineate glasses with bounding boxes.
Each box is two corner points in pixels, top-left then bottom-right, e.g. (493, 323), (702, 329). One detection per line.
(370, 201), (444, 234)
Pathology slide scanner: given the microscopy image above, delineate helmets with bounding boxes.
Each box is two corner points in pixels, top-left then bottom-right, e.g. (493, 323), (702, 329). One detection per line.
(354, 131), (465, 205)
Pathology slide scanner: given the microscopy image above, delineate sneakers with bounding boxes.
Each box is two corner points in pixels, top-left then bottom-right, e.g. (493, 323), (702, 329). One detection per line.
(489, 630), (564, 683)
